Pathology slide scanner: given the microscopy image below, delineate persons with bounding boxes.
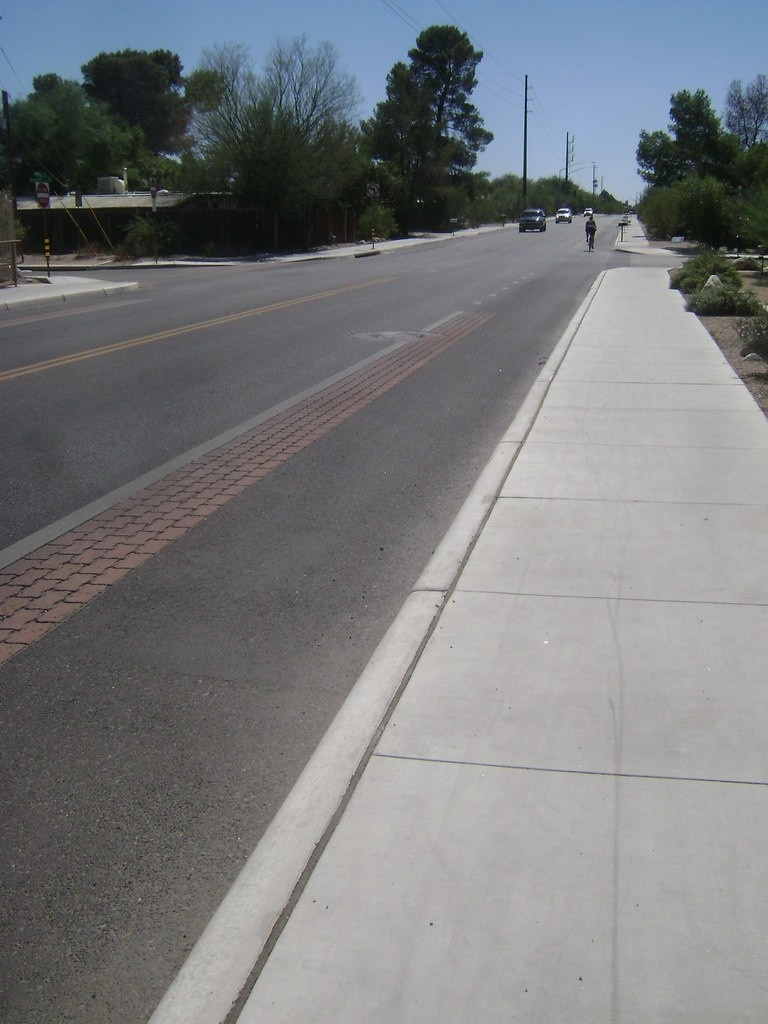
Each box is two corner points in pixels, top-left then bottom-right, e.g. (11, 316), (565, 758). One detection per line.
(585, 217), (597, 249)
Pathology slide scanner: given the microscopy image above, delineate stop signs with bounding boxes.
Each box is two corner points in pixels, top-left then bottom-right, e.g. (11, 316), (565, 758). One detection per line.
(35, 183), (51, 209)
(150, 177), (158, 198)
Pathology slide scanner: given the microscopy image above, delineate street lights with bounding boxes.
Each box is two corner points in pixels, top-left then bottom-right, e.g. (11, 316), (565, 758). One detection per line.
(559, 162), (597, 182)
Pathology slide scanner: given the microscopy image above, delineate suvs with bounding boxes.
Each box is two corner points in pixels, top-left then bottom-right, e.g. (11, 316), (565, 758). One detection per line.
(519, 209), (546, 232)
(555, 208), (573, 224)
(584, 208), (593, 217)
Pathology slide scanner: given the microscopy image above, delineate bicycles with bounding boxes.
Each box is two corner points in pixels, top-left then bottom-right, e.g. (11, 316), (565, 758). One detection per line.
(585, 229), (596, 251)
